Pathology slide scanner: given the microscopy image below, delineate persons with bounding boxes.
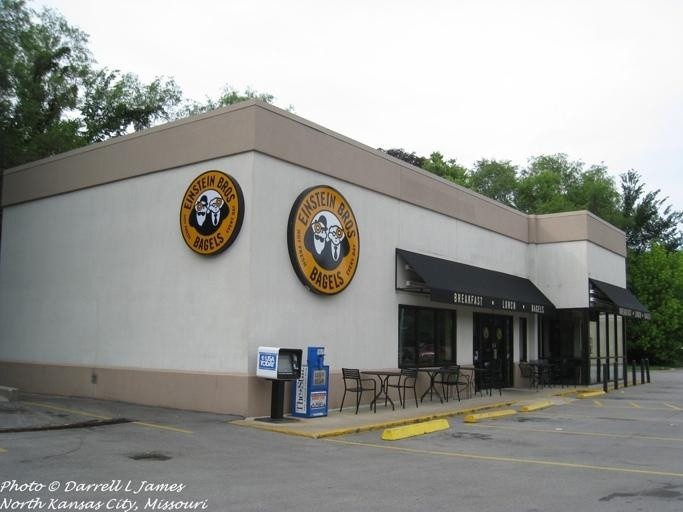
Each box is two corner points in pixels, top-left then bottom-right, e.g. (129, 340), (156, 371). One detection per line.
(189, 195), (208, 235)
(328, 225), (350, 270)
(208, 197), (230, 235)
(304, 215), (328, 270)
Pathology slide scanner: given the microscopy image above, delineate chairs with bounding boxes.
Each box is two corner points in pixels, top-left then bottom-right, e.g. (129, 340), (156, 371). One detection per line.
(339, 358), (503, 414)
(517, 357), (581, 392)
